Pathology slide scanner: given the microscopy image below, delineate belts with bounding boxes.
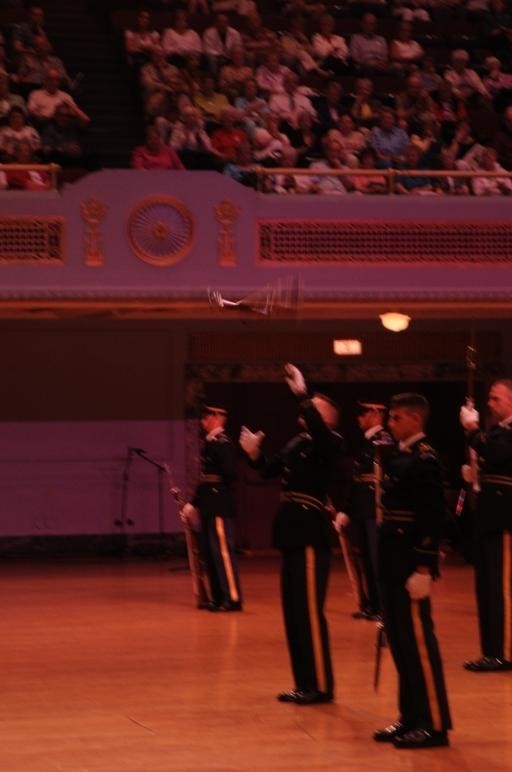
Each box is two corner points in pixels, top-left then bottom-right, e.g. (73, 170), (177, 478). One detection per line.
(199, 472), (223, 483)
(479, 472), (512, 487)
(279, 491), (324, 511)
(382, 507), (418, 523)
(352, 473), (388, 482)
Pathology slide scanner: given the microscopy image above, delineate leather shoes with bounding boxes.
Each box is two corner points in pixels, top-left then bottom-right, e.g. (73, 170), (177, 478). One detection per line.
(296, 693), (331, 704)
(464, 656), (512, 671)
(373, 720), (413, 742)
(391, 726), (449, 748)
(279, 689), (304, 702)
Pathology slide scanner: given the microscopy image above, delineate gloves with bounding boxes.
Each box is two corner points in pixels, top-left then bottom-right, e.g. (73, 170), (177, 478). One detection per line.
(239, 425), (265, 462)
(404, 570), (432, 600)
(283, 363), (307, 396)
(179, 504), (195, 523)
(458, 405), (480, 430)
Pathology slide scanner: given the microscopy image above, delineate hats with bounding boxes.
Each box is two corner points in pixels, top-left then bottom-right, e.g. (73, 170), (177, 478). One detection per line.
(358, 402), (386, 409)
(201, 401), (228, 415)
